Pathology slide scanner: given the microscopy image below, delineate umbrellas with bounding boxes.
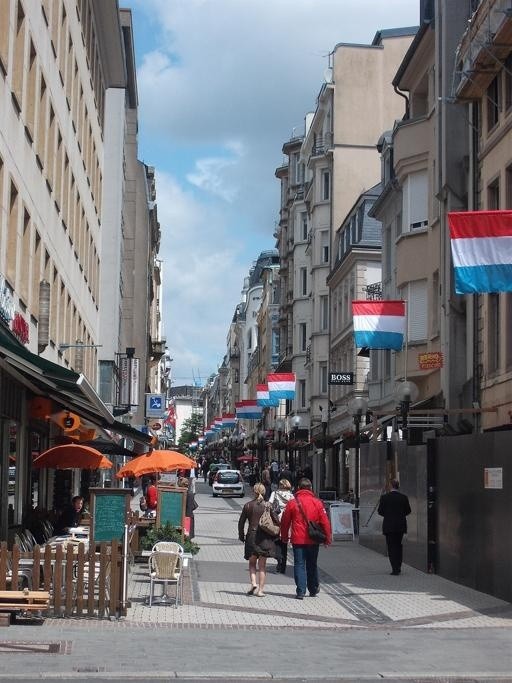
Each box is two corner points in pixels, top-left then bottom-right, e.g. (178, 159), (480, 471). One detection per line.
(33, 444), (112, 495)
(114, 449), (199, 476)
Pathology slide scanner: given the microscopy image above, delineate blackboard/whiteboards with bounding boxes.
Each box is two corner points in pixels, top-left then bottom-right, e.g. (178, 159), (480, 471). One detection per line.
(318, 491), (336, 501)
(330, 504), (354, 535)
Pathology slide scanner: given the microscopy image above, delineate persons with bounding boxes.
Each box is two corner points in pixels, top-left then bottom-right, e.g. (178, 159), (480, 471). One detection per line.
(279, 477), (333, 600)
(52, 493), (88, 539)
(127, 449), (313, 542)
(268, 477), (294, 574)
(236, 480), (281, 597)
(147, 422), (165, 438)
(376, 478), (412, 576)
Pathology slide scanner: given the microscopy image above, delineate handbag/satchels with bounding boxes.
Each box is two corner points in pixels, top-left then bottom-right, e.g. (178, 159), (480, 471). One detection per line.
(306, 519), (327, 543)
(259, 511), (281, 536)
(140, 497), (149, 511)
(272, 498), (281, 514)
(191, 491), (198, 510)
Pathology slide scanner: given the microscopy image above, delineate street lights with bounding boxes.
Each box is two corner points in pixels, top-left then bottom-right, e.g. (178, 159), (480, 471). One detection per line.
(395, 380), (420, 446)
(347, 395), (370, 530)
(202, 414), (303, 490)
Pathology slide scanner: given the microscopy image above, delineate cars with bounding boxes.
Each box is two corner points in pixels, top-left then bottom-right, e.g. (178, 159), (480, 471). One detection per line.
(212, 468), (246, 498)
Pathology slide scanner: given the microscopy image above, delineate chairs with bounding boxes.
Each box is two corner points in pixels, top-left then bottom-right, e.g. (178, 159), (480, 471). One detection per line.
(0, 517), (112, 608)
(141, 540), (192, 608)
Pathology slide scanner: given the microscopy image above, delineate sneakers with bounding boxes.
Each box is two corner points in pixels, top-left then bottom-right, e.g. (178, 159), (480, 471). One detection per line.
(248, 584), (266, 596)
(276, 558), (286, 573)
(390, 567), (401, 575)
(296, 586), (320, 598)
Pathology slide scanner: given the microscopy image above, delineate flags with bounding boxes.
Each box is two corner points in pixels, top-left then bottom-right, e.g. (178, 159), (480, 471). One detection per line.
(447, 208), (512, 292)
(351, 299), (405, 351)
(187, 399), (270, 449)
(256, 384), (279, 406)
(266, 372), (296, 399)
